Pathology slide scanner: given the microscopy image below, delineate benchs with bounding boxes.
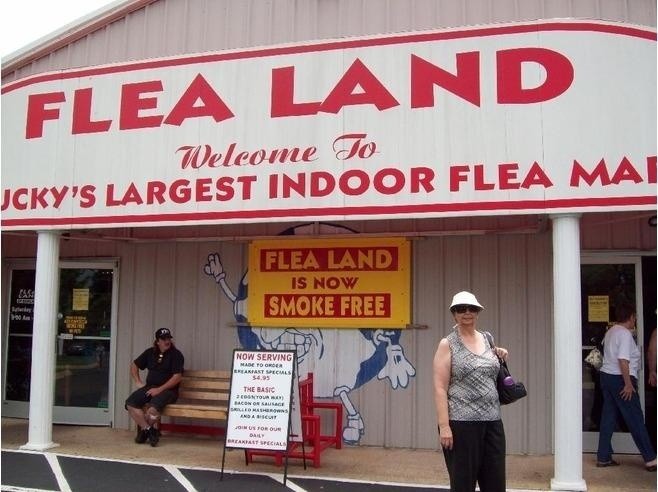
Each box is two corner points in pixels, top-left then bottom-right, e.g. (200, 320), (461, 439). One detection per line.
(144, 370), (232, 440)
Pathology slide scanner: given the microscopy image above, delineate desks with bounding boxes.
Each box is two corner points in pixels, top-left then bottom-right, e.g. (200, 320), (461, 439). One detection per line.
(54, 357), (99, 406)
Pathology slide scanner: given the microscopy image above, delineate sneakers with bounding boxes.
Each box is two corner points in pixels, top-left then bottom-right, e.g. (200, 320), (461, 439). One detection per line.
(135, 428), (149, 444)
(148, 426), (162, 447)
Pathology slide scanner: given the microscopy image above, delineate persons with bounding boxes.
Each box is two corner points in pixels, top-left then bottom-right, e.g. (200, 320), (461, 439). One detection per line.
(123, 327), (185, 448)
(645, 326), (656, 389)
(431, 290), (508, 492)
(595, 301), (657, 472)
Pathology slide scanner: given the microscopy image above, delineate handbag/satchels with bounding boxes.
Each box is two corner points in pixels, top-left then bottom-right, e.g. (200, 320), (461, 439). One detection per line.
(496, 366), (527, 405)
(584, 343), (603, 372)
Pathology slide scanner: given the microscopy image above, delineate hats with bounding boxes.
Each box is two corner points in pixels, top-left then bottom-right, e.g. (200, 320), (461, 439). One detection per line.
(449, 291), (484, 311)
(155, 328), (173, 339)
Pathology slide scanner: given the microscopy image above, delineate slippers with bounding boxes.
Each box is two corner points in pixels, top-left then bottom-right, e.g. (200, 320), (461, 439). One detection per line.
(597, 460), (618, 467)
(647, 464), (657, 471)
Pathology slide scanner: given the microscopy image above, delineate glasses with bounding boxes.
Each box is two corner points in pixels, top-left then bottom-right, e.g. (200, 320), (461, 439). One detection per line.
(455, 306), (477, 313)
(158, 354), (164, 365)
